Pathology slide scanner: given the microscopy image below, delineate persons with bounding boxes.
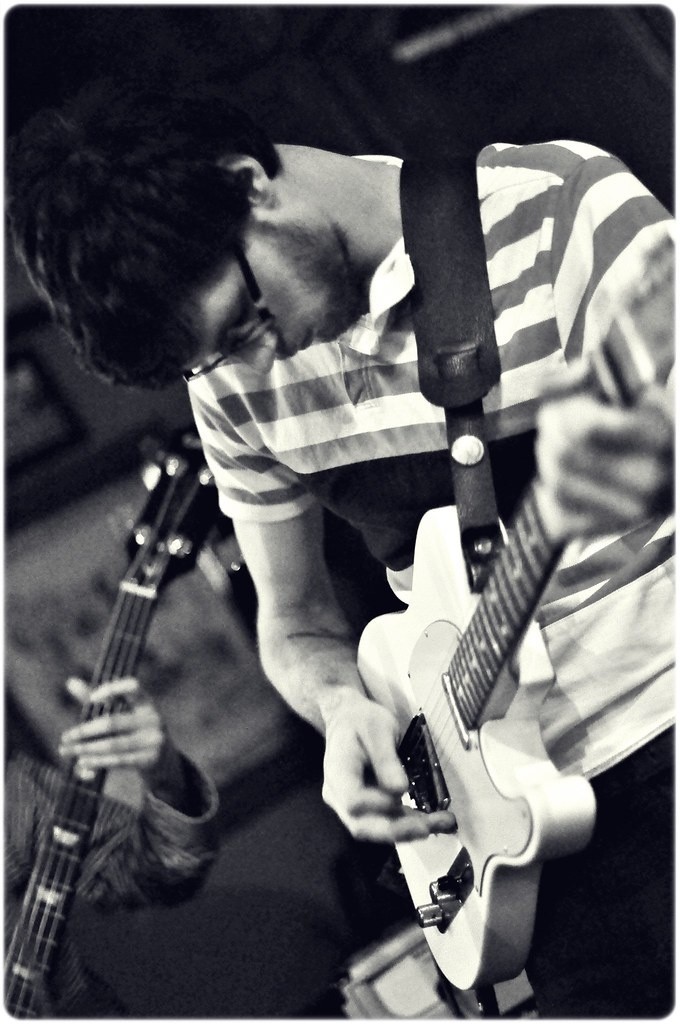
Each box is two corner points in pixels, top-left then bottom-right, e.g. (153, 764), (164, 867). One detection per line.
(6, 676), (223, 1018)
(5, 80), (674, 1019)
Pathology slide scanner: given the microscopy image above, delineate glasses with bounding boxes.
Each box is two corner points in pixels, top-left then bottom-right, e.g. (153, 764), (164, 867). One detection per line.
(183, 237), (276, 380)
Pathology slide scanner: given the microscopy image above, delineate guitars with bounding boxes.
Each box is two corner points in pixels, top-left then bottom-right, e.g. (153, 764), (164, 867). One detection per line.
(4, 429), (219, 1020)
(354, 233), (679, 998)
